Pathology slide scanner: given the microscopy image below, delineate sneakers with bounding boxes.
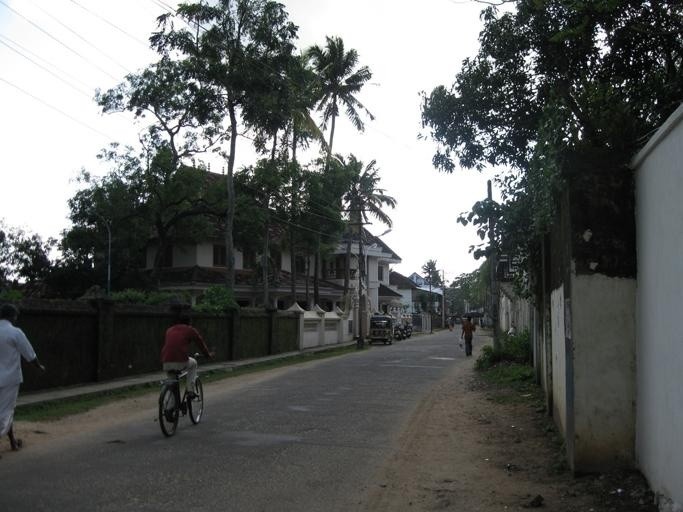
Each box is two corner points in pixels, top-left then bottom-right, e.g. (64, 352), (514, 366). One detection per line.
(163, 409), (174, 423)
(187, 391), (200, 400)
(11, 439), (23, 451)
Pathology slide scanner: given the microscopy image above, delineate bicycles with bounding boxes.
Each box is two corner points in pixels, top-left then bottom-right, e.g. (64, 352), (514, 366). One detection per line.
(395, 320), (413, 341)
(153, 352), (204, 438)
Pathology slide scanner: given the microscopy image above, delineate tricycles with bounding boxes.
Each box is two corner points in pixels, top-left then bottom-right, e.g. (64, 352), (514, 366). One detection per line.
(367, 312), (394, 345)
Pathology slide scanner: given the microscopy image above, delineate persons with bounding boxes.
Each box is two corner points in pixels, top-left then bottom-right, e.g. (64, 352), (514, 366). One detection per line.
(163, 315), (211, 422)
(461, 317), (475, 356)
(0, 304), (45, 450)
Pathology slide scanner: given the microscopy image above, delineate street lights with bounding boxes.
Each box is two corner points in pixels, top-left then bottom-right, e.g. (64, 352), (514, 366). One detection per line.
(337, 228), (393, 336)
